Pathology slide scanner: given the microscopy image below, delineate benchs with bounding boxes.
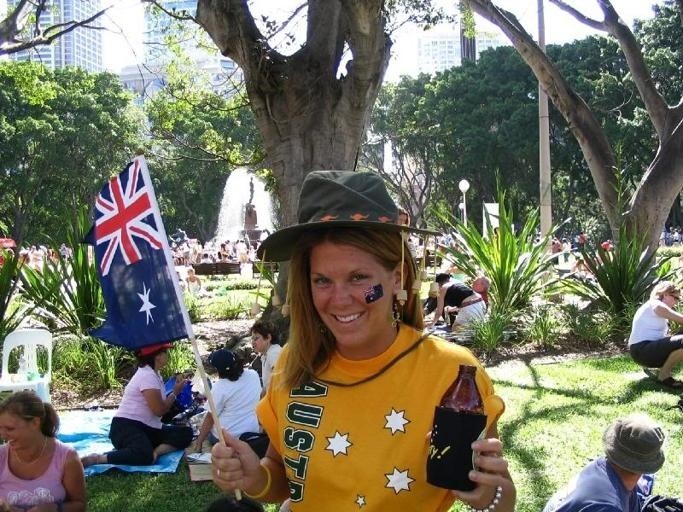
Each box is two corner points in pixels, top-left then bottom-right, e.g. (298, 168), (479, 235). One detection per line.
(252, 261), (280, 279)
(416, 257), (444, 270)
(191, 263), (242, 279)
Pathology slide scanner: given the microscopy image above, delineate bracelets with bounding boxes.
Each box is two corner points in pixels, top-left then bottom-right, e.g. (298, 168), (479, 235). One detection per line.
(463, 486), (503, 512)
(171, 393), (176, 400)
(243, 464), (271, 499)
(55, 499), (64, 512)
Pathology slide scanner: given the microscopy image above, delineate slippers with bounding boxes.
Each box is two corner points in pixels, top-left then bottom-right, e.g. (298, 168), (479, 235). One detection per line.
(655, 374), (683, 390)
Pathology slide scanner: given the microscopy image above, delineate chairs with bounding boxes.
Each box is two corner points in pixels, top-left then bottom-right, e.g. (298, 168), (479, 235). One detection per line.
(0, 329), (53, 402)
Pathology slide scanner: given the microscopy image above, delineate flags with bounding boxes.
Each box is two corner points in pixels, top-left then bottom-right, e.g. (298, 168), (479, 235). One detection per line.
(78, 155), (193, 349)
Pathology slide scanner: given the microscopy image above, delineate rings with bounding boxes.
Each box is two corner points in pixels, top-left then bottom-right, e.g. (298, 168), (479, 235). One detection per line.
(217, 469), (220, 477)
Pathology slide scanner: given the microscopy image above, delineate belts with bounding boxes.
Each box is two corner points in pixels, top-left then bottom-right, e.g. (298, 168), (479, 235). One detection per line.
(460, 297), (484, 308)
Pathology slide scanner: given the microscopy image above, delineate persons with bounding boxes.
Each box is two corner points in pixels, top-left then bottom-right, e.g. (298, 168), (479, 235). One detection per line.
(659, 225), (683, 247)
(425, 272), (487, 331)
(472, 277), (491, 310)
(601, 240), (610, 251)
(194, 349), (261, 453)
(81, 343), (193, 467)
(0, 391), (87, 512)
(172, 239), (262, 265)
(6, 241), (73, 274)
(561, 238), (571, 263)
(186, 268), (201, 293)
(212, 170), (517, 512)
(543, 412), (666, 512)
(578, 232), (585, 250)
(408, 230), (467, 257)
(628, 280), (683, 389)
(249, 319), (283, 396)
(554, 238), (561, 250)
(552, 241), (558, 253)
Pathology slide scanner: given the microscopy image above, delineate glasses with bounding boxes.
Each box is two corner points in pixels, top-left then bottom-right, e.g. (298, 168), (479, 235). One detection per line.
(669, 294), (681, 302)
(250, 335), (263, 340)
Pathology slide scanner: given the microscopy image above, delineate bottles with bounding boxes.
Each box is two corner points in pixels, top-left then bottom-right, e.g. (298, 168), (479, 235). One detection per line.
(425, 364), (488, 491)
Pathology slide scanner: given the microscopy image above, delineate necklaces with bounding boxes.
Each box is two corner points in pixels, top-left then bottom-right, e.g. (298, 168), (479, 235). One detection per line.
(14, 435), (47, 465)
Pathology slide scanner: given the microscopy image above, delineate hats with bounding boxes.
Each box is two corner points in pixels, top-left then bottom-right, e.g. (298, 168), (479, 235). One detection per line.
(139, 342), (175, 357)
(249, 170), (444, 318)
(602, 415), (665, 476)
(208, 348), (237, 370)
